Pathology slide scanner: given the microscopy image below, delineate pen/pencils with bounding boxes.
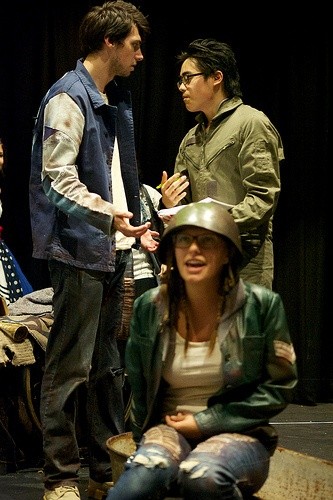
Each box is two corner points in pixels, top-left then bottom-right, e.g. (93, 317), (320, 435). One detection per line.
(156, 173), (181, 189)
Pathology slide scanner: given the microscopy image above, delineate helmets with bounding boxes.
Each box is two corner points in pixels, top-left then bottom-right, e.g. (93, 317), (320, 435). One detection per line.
(162, 201), (243, 262)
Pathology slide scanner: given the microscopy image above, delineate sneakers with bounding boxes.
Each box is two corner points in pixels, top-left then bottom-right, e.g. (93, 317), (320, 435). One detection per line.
(88, 476), (114, 500)
(43, 478), (80, 500)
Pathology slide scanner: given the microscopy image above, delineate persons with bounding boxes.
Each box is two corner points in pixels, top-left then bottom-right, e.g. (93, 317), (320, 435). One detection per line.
(160, 40), (285, 291)
(107, 201), (298, 500)
(29, 0), (150, 500)
(112, 176), (171, 435)
(1, 136), (59, 480)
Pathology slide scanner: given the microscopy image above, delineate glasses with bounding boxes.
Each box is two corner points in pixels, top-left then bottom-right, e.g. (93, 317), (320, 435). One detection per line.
(175, 232), (225, 249)
(177, 72), (208, 89)
